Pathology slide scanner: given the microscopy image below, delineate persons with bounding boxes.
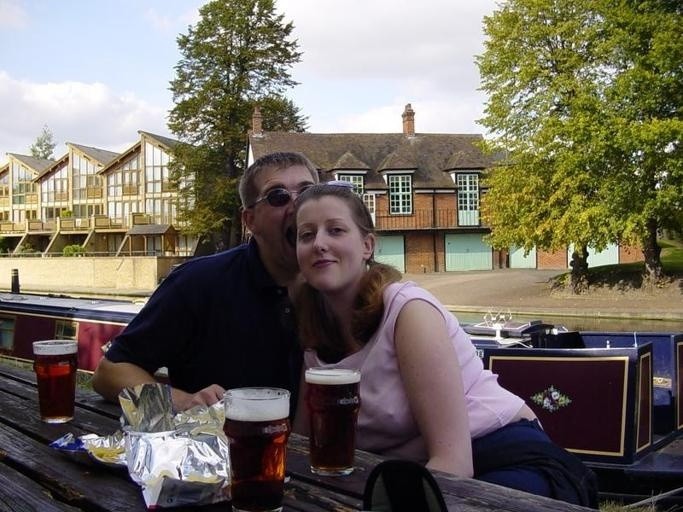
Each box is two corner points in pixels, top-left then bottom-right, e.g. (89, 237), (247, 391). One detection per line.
(88, 150), (322, 416)
(285, 180), (601, 510)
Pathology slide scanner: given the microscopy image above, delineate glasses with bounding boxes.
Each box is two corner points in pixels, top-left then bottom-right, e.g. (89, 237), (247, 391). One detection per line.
(298, 179), (354, 195)
(245, 184), (314, 209)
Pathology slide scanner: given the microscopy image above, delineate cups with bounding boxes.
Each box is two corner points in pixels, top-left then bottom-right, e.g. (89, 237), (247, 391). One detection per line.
(32, 340), (77, 424)
(223, 366), (361, 511)
